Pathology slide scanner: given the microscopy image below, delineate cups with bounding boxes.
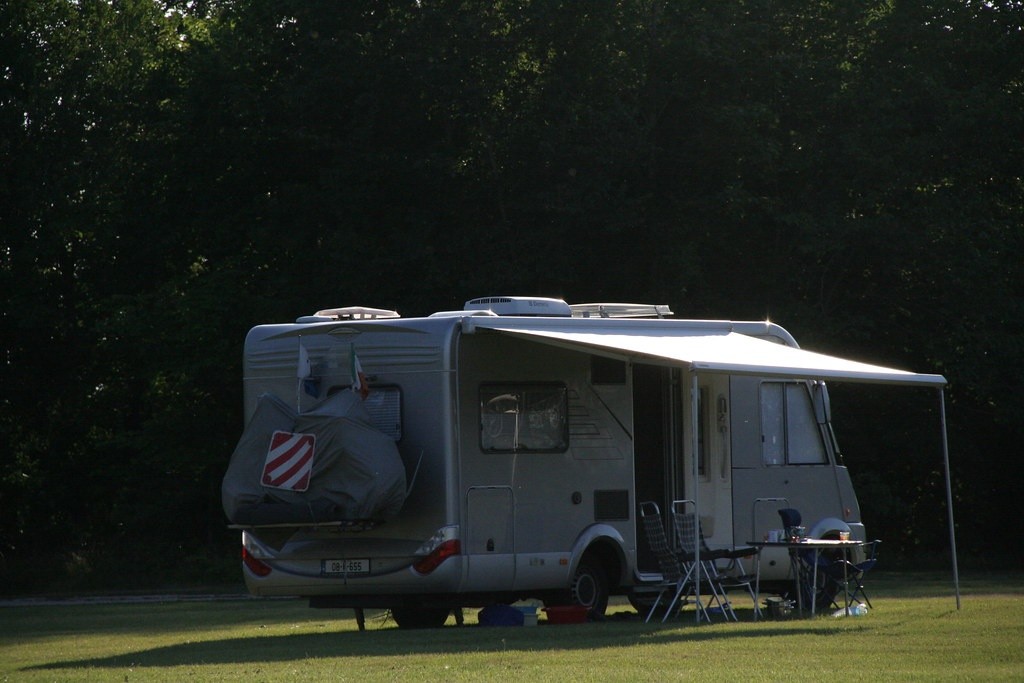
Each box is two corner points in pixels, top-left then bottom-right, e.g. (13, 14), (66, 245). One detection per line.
(768, 529), (785, 542)
(840, 531), (849, 540)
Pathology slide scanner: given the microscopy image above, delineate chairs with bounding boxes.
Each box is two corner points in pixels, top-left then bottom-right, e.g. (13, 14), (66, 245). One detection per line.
(639, 497), (881, 625)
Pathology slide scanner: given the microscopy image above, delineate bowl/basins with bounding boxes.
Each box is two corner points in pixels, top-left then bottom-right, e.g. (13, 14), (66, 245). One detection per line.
(523, 614), (541, 626)
(512, 607), (538, 614)
(541, 606), (587, 624)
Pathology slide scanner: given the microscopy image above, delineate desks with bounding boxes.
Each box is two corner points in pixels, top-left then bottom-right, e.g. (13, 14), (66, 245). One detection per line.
(744, 538), (862, 621)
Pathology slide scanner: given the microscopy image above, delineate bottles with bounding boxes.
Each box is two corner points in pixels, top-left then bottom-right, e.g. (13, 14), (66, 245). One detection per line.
(832, 604), (867, 616)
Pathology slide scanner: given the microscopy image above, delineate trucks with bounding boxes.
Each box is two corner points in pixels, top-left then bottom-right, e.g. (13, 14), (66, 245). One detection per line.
(241, 297), (949, 628)
